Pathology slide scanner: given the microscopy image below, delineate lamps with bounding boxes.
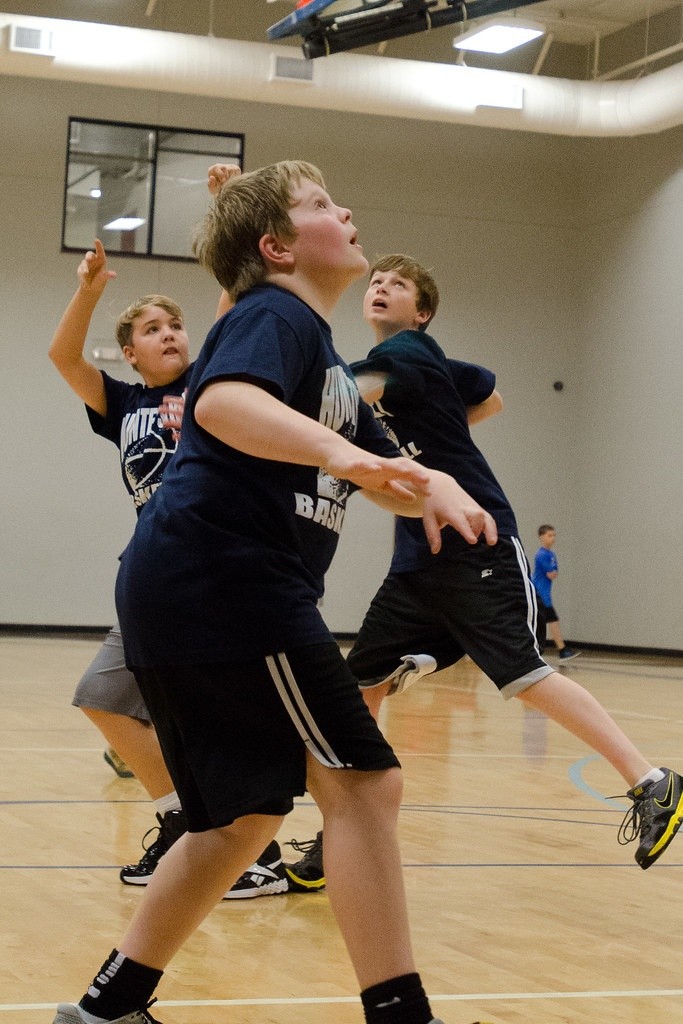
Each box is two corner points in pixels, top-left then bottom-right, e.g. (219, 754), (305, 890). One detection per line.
(452, 17), (548, 54)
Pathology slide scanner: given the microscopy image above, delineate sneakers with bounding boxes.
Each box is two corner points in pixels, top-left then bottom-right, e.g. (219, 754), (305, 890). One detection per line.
(120, 809), (188, 885)
(52, 997), (164, 1024)
(282, 830), (326, 889)
(222, 839), (289, 898)
(557, 648), (581, 661)
(605, 767), (683, 870)
(104, 745), (135, 778)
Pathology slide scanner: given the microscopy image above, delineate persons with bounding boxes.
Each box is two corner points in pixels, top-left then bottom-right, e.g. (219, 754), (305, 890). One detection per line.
(48, 163), (290, 901)
(53, 158), (499, 1024)
(533, 523), (575, 658)
(285, 252), (683, 893)
